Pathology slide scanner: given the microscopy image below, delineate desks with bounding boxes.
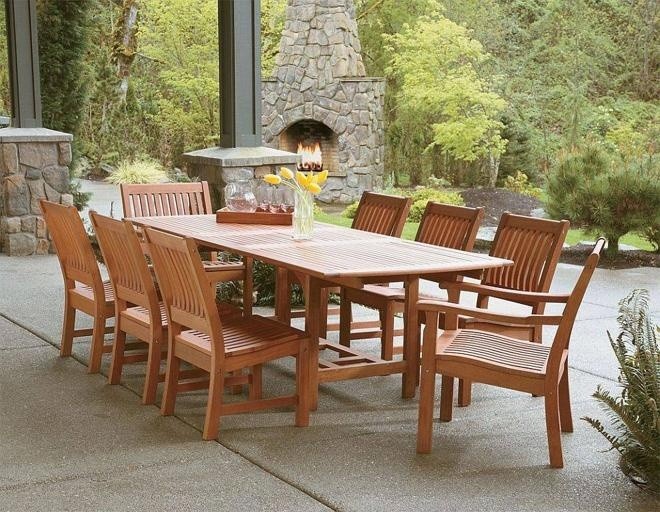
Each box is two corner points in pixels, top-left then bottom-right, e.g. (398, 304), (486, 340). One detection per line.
(121, 214), (515, 412)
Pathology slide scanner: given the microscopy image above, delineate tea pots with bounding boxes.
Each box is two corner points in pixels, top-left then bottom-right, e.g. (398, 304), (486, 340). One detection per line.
(225, 179), (257, 213)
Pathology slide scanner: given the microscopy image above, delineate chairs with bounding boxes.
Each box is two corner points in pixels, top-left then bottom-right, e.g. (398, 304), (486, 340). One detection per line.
(418, 211), (570, 407)
(37, 197), (158, 374)
(415, 237), (607, 469)
(120, 181), (245, 303)
(340, 200), (485, 376)
(88, 207), (244, 405)
(288, 188), (412, 350)
(142, 226), (311, 440)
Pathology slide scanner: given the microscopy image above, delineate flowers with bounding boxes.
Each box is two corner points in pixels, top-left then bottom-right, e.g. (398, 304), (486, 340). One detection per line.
(263, 167), (329, 232)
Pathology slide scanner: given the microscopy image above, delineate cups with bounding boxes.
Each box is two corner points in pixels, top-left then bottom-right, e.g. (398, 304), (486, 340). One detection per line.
(261, 186), (292, 213)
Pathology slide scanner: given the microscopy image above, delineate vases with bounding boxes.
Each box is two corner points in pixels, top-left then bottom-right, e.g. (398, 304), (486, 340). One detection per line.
(292, 189), (313, 241)
